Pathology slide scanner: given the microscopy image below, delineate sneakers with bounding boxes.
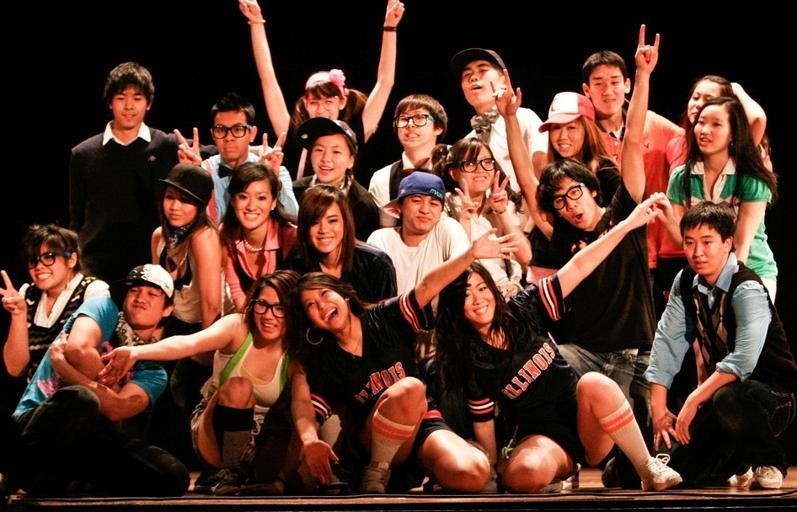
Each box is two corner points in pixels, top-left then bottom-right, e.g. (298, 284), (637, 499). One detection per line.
(637, 454), (683, 492)
(540, 463), (582, 494)
(727, 465), (752, 488)
(471, 442), (499, 495)
(206, 464), (243, 495)
(4, 485), (30, 511)
(313, 473), (348, 495)
(497, 446), (514, 483)
(363, 461), (392, 494)
(752, 465), (783, 489)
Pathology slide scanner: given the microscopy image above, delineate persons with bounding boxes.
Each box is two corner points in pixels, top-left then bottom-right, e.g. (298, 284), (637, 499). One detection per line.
(0, 0), (797, 503)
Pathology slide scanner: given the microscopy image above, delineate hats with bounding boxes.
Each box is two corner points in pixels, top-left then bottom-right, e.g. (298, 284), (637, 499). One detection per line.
(382, 172), (446, 220)
(538, 92), (595, 134)
(295, 116), (359, 155)
(123, 264), (175, 298)
(159, 164), (214, 205)
(451, 47), (507, 80)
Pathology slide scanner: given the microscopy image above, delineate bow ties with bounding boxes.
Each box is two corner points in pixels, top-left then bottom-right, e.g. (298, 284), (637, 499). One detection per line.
(219, 165), (236, 177)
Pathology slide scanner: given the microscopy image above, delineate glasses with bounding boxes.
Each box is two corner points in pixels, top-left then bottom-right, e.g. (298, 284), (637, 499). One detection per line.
(303, 96), (340, 111)
(26, 252), (67, 270)
(454, 158), (496, 173)
(212, 125), (251, 139)
(552, 182), (585, 210)
(394, 114), (434, 128)
(250, 300), (288, 318)
